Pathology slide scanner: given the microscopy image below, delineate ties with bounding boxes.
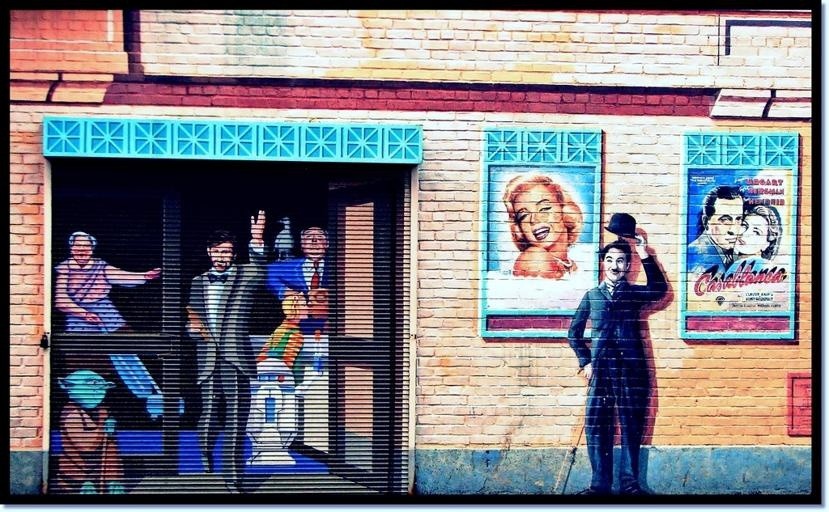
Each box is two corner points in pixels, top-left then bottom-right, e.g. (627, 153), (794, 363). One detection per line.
(310, 260), (321, 289)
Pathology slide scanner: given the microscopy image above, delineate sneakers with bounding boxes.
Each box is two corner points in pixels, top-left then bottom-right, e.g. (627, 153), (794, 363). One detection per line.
(574, 483), (651, 499)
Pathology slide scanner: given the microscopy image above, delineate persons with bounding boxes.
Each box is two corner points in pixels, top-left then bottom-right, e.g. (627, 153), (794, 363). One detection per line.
(306, 286), (331, 373)
(716, 200), (787, 275)
(54, 230), (188, 424)
(567, 233), (669, 494)
(500, 166), (581, 283)
(266, 222), (330, 334)
(184, 207), (268, 495)
(250, 290), (311, 388)
(683, 182), (747, 284)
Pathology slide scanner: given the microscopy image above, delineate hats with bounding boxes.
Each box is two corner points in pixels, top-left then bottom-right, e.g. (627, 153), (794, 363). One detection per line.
(605, 212), (641, 239)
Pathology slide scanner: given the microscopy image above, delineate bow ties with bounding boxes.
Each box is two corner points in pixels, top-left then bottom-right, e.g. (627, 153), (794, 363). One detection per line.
(207, 273), (229, 285)
